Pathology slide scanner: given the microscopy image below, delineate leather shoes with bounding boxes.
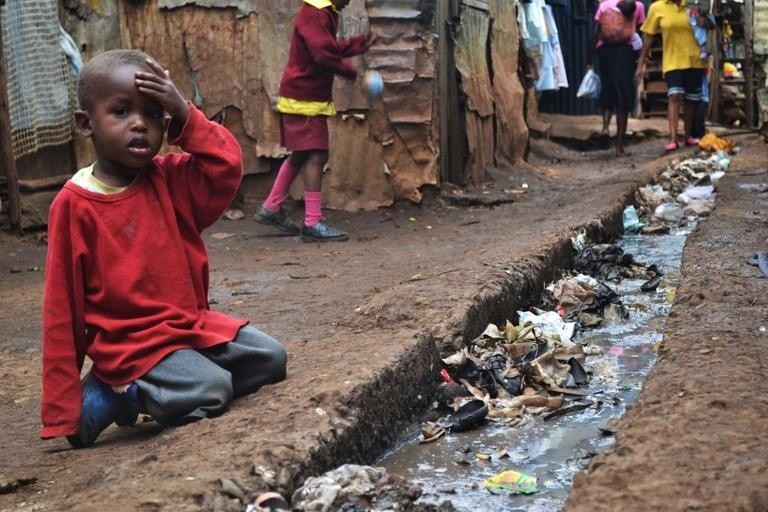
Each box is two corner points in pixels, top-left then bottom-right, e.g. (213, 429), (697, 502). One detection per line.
(302, 222), (349, 242)
(255, 205), (301, 234)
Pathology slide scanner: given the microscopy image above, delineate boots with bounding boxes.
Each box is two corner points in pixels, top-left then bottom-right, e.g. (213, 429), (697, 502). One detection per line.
(66, 367), (145, 448)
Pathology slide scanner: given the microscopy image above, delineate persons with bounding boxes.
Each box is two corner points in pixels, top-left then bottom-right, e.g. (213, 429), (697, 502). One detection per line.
(587, 0), (646, 158)
(685, 0), (711, 60)
(634, 0), (717, 150)
(38, 45), (285, 451)
(252, 1), (381, 242)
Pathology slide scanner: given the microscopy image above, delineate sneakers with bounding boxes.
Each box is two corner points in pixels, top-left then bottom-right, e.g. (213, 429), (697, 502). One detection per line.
(665, 143), (679, 151)
(688, 137), (700, 144)
